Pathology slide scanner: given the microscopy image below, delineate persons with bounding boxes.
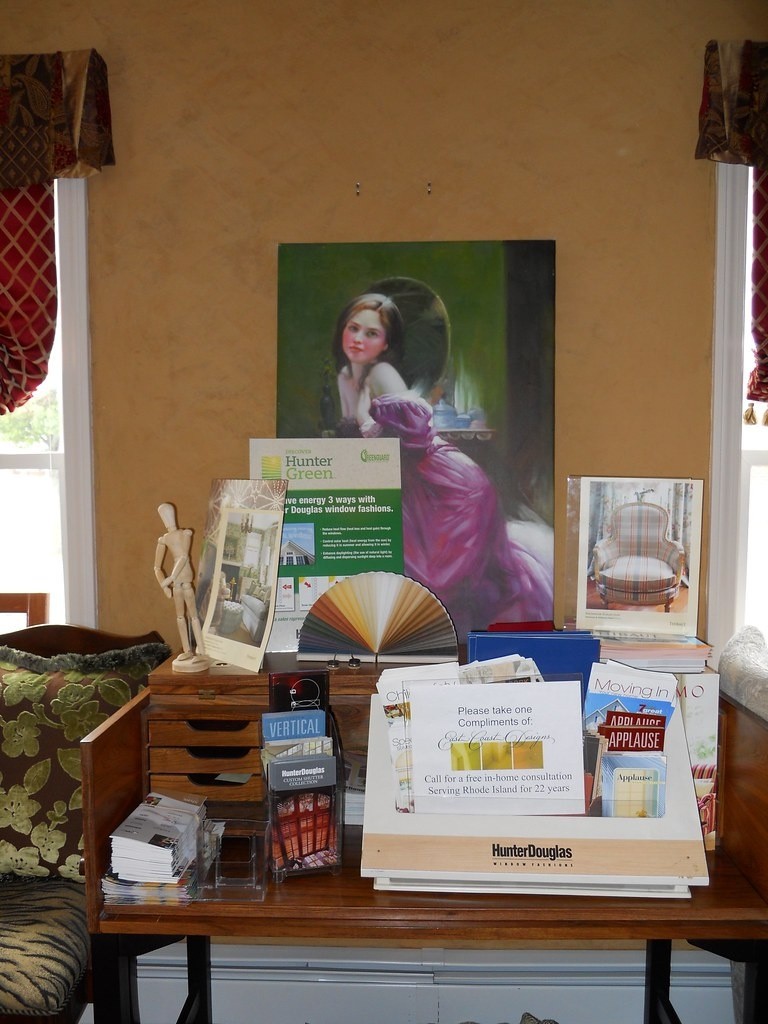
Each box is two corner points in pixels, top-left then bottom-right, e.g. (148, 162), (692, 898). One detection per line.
(153, 503), (208, 663)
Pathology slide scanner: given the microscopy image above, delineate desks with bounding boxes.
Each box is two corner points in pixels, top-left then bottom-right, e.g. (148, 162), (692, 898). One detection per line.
(79, 647), (768, 1024)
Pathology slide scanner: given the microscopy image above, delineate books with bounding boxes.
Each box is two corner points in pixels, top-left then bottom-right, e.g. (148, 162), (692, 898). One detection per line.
(591, 629), (713, 674)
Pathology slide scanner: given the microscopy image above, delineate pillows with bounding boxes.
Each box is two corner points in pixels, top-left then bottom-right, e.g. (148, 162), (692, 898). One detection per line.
(0, 641), (173, 886)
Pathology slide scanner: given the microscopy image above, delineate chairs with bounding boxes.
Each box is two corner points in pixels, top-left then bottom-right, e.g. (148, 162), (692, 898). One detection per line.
(0, 623), (166, 1024)
(589, 501), (687, 612)
